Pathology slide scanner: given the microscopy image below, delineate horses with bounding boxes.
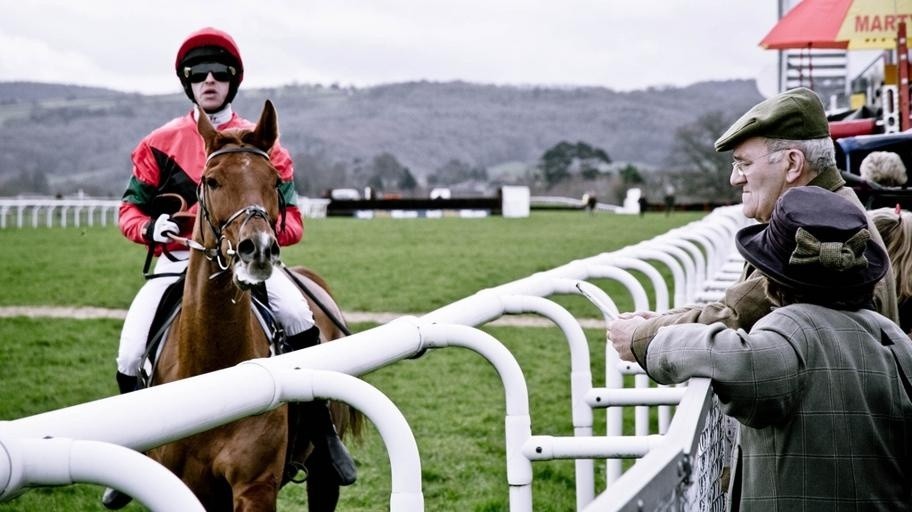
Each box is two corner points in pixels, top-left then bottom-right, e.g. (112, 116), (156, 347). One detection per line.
(142, 98), (369, 512)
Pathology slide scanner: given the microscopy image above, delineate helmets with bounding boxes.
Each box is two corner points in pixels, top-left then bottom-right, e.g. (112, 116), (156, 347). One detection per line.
(175, 26), (244, 102)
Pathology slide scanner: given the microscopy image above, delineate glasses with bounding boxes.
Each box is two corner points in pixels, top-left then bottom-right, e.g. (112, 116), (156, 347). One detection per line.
(183, 61), (234, 81)
(731, 146), (789, 175)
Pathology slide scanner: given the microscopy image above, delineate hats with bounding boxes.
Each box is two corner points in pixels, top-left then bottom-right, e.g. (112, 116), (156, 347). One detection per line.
(715, 86), (831, 152)
(735, 186), (889, 297)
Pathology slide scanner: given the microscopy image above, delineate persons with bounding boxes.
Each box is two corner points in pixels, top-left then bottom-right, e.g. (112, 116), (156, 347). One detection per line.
(644, 186), (912, 512)
(100, 28), (357, 508)
(607, 87), (901, 370)
(868, 204), (912, 341)
(859, 150), (908, 187)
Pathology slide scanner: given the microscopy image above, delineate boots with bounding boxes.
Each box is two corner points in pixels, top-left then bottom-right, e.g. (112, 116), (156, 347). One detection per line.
(285, 326), (358, 485)
(102, 371), (156, 510)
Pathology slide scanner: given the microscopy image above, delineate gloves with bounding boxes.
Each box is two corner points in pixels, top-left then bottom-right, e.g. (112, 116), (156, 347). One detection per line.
(143, 213), (182, 245)
